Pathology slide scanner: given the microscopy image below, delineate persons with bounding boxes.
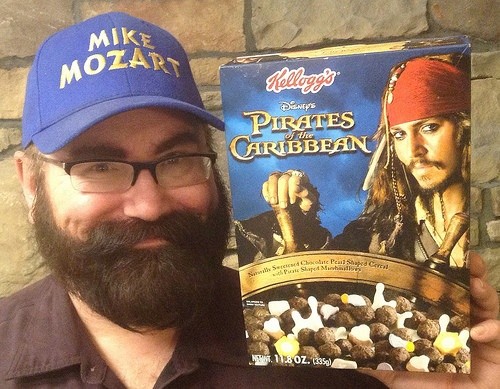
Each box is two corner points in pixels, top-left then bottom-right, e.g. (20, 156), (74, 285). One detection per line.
(260, 55), (472, 284)
(0, 11), (500, 389)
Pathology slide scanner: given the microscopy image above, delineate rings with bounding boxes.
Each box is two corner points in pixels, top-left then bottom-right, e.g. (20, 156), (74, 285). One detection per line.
(269, 168), (284, 178)
(292, 169), (305, 179)
(282, 171), (291, 177)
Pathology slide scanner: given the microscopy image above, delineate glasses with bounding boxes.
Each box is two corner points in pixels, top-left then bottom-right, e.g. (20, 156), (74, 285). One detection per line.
(27, 149), (218, 193)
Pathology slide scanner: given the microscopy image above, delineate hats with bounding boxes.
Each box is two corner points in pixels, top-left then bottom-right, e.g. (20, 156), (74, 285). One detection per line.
(19, 10), (225, 154)
